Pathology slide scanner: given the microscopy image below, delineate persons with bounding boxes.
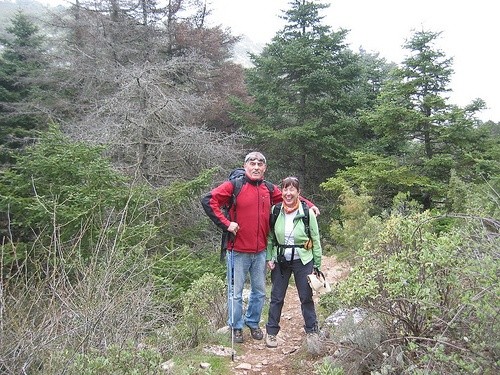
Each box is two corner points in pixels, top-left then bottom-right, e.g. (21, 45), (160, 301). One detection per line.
(200, 151), (320, 343)
(264, 177), (322, 348)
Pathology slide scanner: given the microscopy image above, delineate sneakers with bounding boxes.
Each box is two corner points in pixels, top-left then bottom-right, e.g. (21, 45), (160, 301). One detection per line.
(245, 322), (263, 340)
(265, 332), (278, 348)
(231, 327), (243, 343)
(306, 332), (319, 345)
